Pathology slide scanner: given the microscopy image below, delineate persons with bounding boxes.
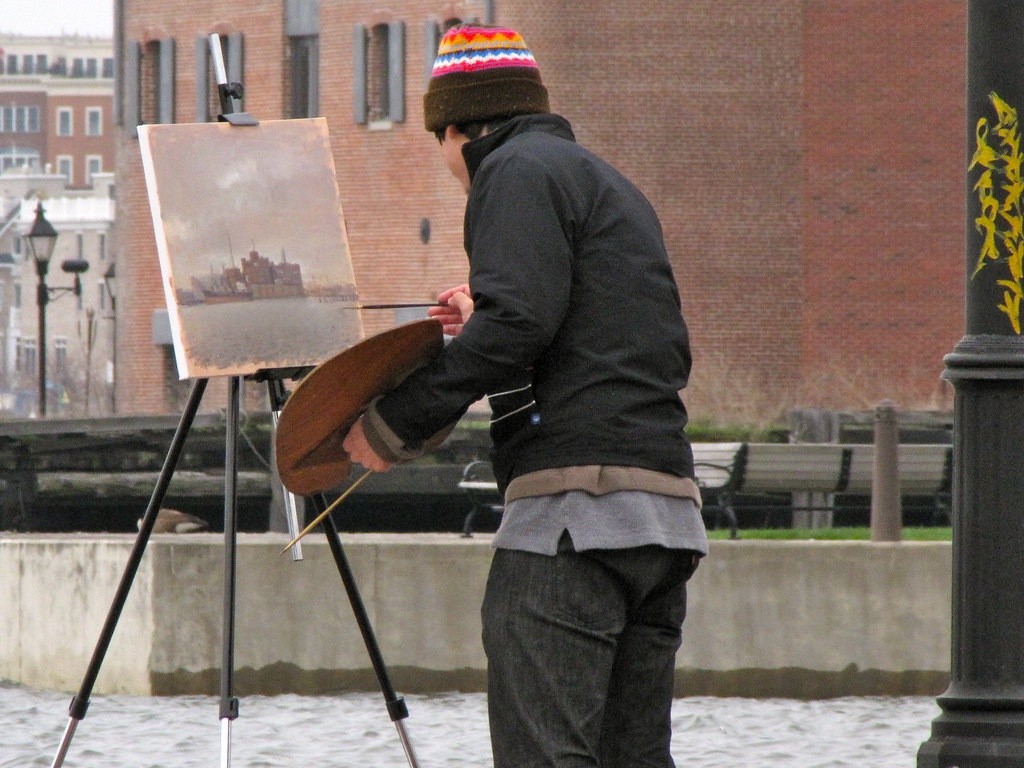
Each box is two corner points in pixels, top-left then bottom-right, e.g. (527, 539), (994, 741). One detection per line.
(340, 22), (710, 768)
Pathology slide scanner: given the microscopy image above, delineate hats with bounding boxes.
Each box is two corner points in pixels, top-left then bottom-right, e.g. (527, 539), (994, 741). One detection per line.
(423, 22), (551, 132)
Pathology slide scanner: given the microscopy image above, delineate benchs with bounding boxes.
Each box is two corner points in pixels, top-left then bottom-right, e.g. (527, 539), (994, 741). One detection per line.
(459, 443), (954, 541)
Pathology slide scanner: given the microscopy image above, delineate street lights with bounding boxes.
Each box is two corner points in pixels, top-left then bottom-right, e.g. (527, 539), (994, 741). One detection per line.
(104, 262), (116, 413)
(22, 201), (59, 414)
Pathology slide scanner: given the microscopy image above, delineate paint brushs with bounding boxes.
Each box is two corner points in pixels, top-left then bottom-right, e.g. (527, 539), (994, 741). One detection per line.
(280, 470), (374, 560)
(342, 303), (451, 312)
